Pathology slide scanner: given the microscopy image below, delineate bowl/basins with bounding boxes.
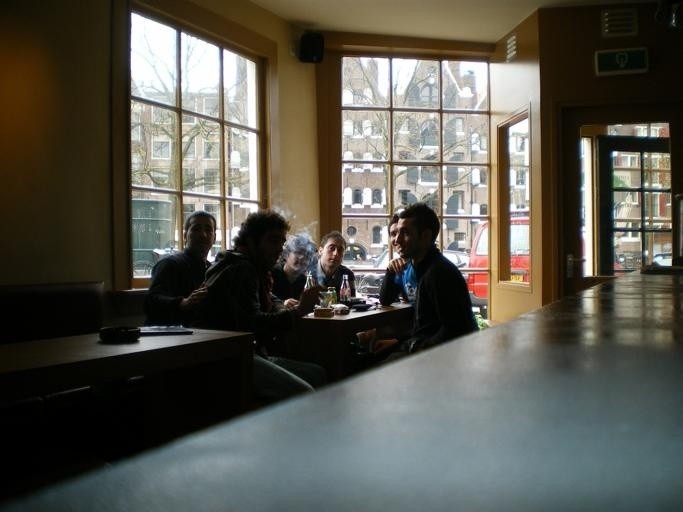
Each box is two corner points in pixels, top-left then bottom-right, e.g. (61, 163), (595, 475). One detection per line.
(110, 325), (140, 341)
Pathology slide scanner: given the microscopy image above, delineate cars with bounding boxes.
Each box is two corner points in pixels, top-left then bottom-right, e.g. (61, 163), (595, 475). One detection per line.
(343, 242), (370, 262)
(373, 249), (404, 269)
(619, 251), (647, 268)
(447, 240), (468, 250)
(131, 248), (164, 278)
(653, 253), (672, 266)
(441, 249), (469, 269)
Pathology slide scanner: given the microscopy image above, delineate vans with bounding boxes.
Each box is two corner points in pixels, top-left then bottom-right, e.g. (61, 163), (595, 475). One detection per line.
(466, 217), (628, 317)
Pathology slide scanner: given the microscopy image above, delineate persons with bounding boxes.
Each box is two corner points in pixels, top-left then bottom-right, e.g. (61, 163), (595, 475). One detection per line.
(309, 230), (356, 302)
(181, 209), (328, 401)
(375, 203), (480, 366)
(272, 233), (318, 309)
(144, 208), (217, 327)
(369, 214), (418, 353)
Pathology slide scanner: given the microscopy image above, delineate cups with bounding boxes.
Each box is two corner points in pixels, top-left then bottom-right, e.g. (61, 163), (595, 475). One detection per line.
(318, 292), (333, 308)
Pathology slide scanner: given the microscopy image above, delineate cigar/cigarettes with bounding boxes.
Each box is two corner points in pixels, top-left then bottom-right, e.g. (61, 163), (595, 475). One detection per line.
(314, 280), (316, 288)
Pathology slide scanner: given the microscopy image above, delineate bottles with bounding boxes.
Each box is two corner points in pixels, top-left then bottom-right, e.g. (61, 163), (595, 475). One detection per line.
(327, 287), (337, 304)
(303, 272), (315, 290)
(340, 274), (351, 302)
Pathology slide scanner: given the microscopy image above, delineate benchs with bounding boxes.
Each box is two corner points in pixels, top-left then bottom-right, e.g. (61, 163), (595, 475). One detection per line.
(0, 282), (113, 351)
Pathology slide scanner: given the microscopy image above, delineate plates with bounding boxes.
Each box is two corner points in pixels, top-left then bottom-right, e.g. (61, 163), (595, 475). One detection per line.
(351, 303), (372, 311)
